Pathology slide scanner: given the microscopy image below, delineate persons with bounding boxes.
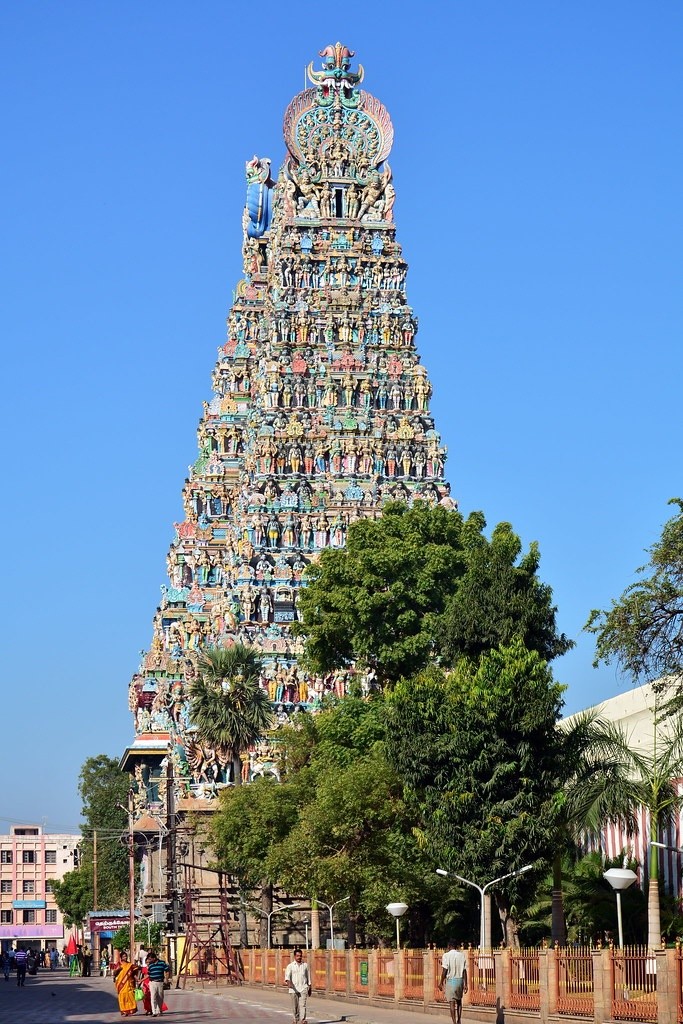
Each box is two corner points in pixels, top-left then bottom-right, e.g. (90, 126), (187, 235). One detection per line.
(130, 169), (455, 792)
(0, 944), (219, 1021)
(284, 950), (312, 1024)
(437, 939), (468, 1024)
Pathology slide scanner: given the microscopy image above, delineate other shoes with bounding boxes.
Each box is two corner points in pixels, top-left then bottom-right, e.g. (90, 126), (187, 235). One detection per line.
(301, 1019), (307, 1024)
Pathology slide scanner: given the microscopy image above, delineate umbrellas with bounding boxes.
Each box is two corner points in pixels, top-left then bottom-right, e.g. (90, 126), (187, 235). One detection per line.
(64, 934), (78, 955)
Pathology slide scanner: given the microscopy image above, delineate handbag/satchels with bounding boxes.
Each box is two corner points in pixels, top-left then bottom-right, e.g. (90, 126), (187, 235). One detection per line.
(135, 989), (145, 1001)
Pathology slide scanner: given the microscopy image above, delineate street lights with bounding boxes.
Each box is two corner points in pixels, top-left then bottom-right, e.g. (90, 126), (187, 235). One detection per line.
(601, 868), (638, 949)
(436, 863), (533, 991)
(242, 901), (301, 949)
(303, 915), (311, 951)
(386, 903), (409, 950)
(298, 894), (351, 951)
